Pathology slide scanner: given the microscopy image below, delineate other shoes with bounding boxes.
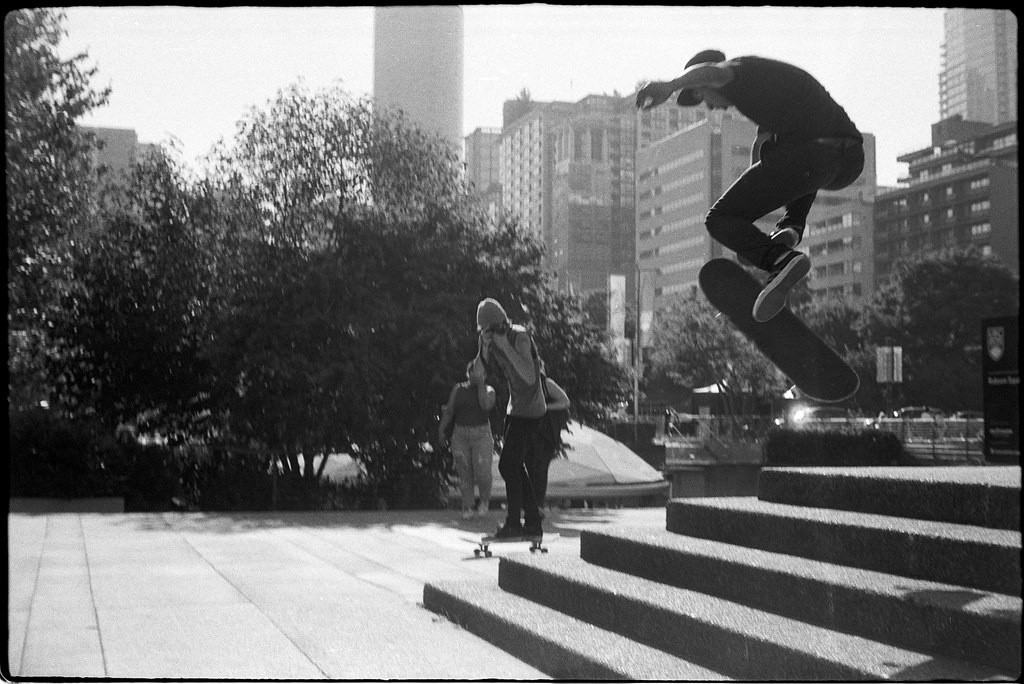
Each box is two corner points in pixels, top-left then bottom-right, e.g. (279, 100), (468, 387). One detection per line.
(494, 521), (543, 537)
(463, 507), (474, 521)
(478, 501), (489, 518)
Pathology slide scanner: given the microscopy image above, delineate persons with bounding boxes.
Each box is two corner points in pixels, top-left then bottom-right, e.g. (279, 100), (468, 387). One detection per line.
(636, 50), (865, 324)
(468, 296), (548, 539)
(518, 355), (571, 520)
(438, 361), (496, 520)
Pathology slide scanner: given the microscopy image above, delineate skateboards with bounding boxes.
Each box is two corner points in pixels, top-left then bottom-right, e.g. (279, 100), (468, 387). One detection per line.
(458, 531), (562, 556)
(699, 258), (861, 404)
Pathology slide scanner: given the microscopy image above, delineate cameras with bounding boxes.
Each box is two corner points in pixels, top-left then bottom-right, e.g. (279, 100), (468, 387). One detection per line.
(488, 322), (506, 335)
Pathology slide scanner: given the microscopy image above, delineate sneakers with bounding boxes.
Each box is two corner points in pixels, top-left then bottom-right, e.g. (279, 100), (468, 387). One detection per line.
(752, 251), (811, 322)
(737, 224), (803, 267)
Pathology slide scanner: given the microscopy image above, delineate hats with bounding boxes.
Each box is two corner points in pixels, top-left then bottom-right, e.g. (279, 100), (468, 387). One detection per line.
(477, 298), (507, 328)
(677, 50), (725, 106)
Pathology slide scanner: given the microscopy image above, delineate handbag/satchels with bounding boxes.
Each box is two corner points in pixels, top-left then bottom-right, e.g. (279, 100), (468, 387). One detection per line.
(442, 405), (457, 439)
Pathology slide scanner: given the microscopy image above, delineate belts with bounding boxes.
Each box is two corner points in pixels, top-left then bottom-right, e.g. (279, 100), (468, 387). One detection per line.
(773, 134), (859, 147)
